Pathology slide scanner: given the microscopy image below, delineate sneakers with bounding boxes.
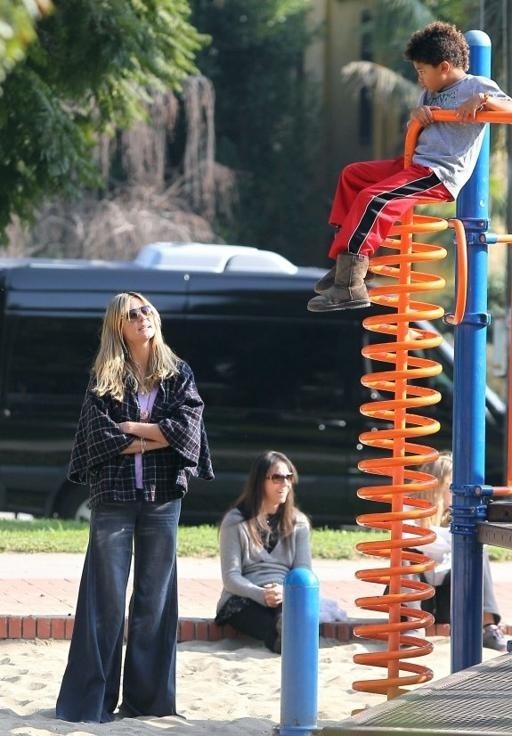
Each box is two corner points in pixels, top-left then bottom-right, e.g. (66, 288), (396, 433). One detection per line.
(483, 625), (507, 651)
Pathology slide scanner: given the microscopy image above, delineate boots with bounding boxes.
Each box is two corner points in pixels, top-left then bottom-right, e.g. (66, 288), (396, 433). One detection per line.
(307, 229), (371, 313)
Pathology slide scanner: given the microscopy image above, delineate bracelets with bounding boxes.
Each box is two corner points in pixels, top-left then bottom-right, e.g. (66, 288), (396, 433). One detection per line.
(480, 91), (489, 108)
(139, 438), (146, 454)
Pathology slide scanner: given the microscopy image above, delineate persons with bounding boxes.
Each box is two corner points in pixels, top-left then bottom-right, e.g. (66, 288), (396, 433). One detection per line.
(53, 292), (215, 724)
(215, 448), (314, 656)
(306, 19), (512, 315)
(379, 450), (510, 652)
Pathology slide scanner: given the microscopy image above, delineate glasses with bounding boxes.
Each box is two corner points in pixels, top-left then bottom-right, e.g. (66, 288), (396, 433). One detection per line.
(124, 305), (152, 322)
(264, 473), (295, 484)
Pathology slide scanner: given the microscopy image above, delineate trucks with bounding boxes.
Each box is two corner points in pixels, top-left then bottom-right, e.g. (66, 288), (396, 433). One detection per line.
(2, 243), (297, 267)
(1, 258), (512, 527)
